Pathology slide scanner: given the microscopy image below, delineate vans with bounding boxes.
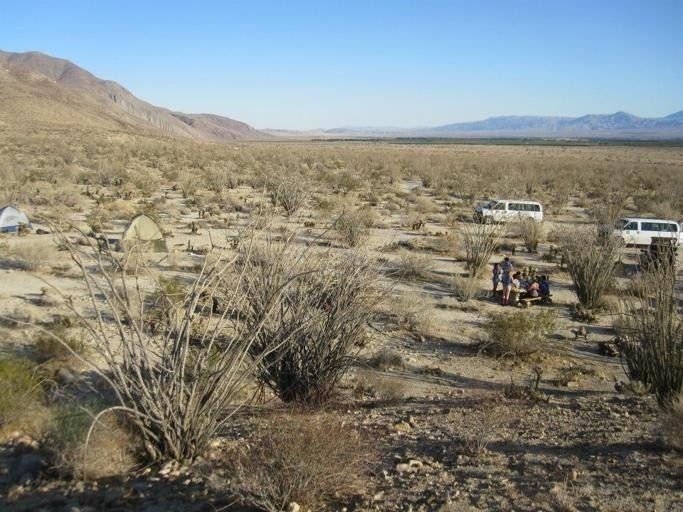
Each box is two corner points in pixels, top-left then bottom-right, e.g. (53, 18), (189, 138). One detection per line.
(597, 217), (683, 249)
(474, 200), (543, 225)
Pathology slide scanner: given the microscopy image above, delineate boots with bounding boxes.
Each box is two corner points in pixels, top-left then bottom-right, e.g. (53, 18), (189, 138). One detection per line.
(501, 298), (509, 306)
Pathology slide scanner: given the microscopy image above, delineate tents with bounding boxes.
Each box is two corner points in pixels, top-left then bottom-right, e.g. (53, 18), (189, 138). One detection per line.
(120, 213), (169, 255)
(0, 205), (33, 235)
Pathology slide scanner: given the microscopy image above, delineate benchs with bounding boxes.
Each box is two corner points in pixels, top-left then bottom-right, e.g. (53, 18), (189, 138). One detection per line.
(519, 294), (553, 308)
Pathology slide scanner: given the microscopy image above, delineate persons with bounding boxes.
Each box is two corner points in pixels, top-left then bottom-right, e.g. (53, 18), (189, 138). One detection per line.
(491, 255), (550, 307)
